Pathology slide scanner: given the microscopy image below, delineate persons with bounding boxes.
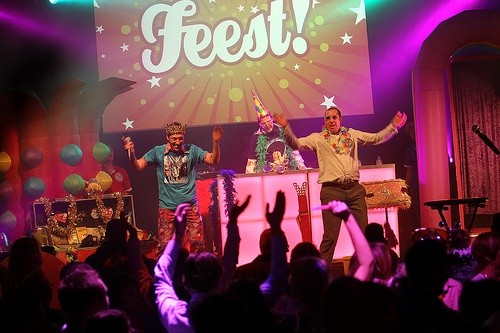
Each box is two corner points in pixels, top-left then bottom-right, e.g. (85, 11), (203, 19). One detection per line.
(251, 88), (292, 164)
(273, 107), (407, 274)
(102, 144), (132, 195)
(122, 122), (224, 261)
(0, 191), (500, 333)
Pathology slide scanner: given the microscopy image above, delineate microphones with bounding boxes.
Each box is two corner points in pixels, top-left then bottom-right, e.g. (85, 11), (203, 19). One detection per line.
(472, 125), (483, 136)
(125, 137), (131, 160)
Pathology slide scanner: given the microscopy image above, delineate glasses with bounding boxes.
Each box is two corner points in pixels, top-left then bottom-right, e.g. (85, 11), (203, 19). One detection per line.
(324, 115), (340, 120)
(258, 120), (272, 127)
